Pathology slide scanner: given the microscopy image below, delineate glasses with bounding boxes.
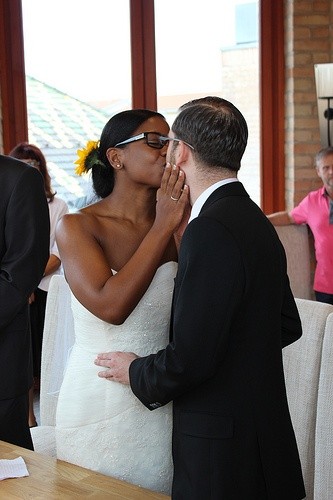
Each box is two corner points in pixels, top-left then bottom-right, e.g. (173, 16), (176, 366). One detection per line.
(114, 132), (166, 150)
(160, 136), (196, 151)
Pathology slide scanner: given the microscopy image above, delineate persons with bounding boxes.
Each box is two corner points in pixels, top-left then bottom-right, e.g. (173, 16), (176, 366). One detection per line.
(96, 96), (307, 500)
(30, 108), (189, 495)
(268, 145), (333, 305)
(8, 142), (69, 428)
(0, 153), (51, 452)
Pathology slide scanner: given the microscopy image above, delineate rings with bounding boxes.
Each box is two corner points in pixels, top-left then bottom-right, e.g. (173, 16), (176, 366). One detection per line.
(171, 197), (178, 200)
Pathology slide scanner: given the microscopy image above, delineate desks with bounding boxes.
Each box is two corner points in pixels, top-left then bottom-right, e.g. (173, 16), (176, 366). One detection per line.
(0, 440), (172, 500)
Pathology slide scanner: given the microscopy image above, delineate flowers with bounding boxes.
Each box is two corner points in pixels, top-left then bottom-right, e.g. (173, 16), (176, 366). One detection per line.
(73, 140), (109, 176)
